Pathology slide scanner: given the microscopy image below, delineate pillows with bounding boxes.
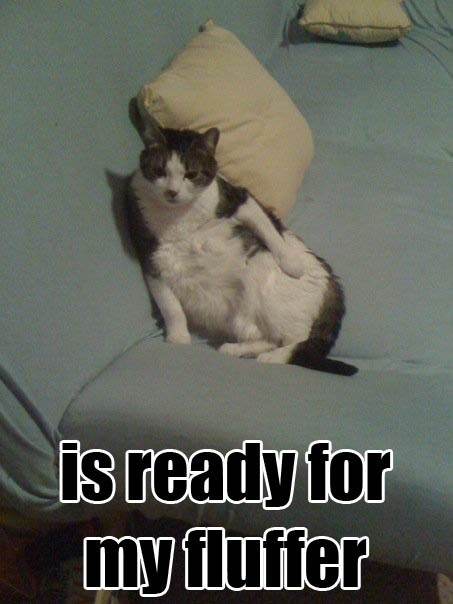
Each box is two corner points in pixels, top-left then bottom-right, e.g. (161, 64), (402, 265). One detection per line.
(135, 19), (314, 228)
(299, 1), (412, 47)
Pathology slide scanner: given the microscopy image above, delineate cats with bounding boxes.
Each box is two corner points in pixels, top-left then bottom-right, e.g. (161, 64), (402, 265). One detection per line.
(121, 95), (360, 378)
